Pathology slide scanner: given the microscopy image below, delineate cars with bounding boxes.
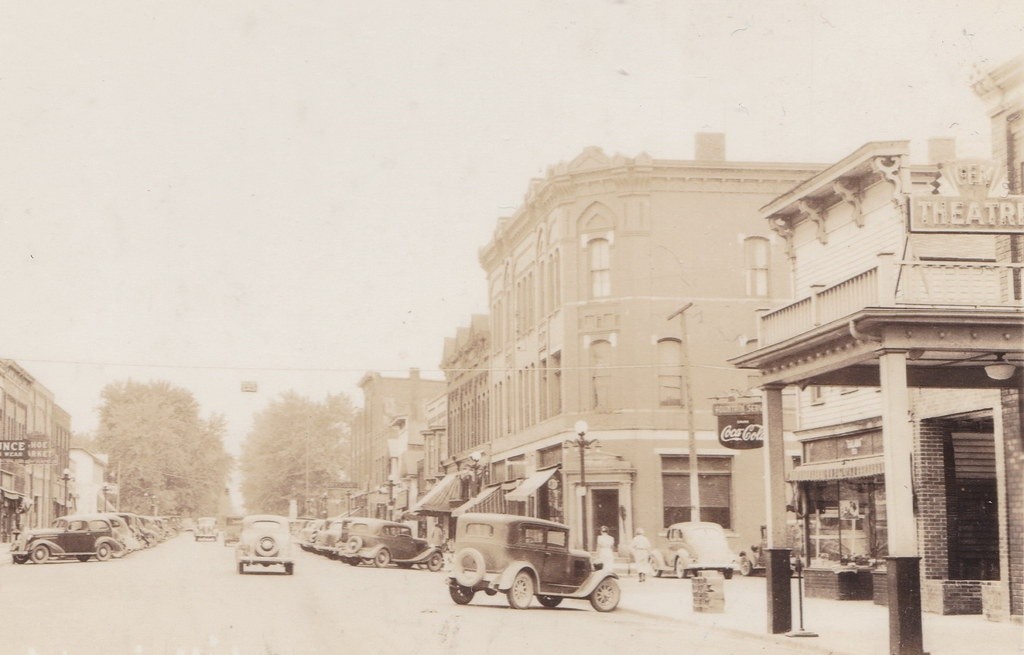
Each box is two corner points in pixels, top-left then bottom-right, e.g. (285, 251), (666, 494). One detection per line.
(649, 522), (734, 578)
(194, 517), (218, 541)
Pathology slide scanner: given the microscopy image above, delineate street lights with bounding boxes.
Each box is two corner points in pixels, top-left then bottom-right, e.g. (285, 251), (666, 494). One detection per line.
(562, 422), (601, 550)
(56, 469), (76, 516)
(465, 453), (487, 492)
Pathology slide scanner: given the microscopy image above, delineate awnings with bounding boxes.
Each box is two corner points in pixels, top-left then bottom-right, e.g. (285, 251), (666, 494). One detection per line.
(414, 473), (462, 515)
(788, 455), (885, 481)
(505, 467), (558, 502)
(451, 486), (504, 518)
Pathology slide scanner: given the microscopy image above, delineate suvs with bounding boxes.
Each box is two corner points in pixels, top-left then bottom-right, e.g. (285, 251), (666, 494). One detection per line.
(298, 518), (443, 572)
(235, 515), (296, 574)
(11, 514), (181, 564)
(446, 513), (621, 612)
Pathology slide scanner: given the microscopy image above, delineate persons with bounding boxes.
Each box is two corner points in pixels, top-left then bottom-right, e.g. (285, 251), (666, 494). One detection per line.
(632, 529), (649, 582)
(595, 526), (614, 572)
(431, 524), (443, 548)
